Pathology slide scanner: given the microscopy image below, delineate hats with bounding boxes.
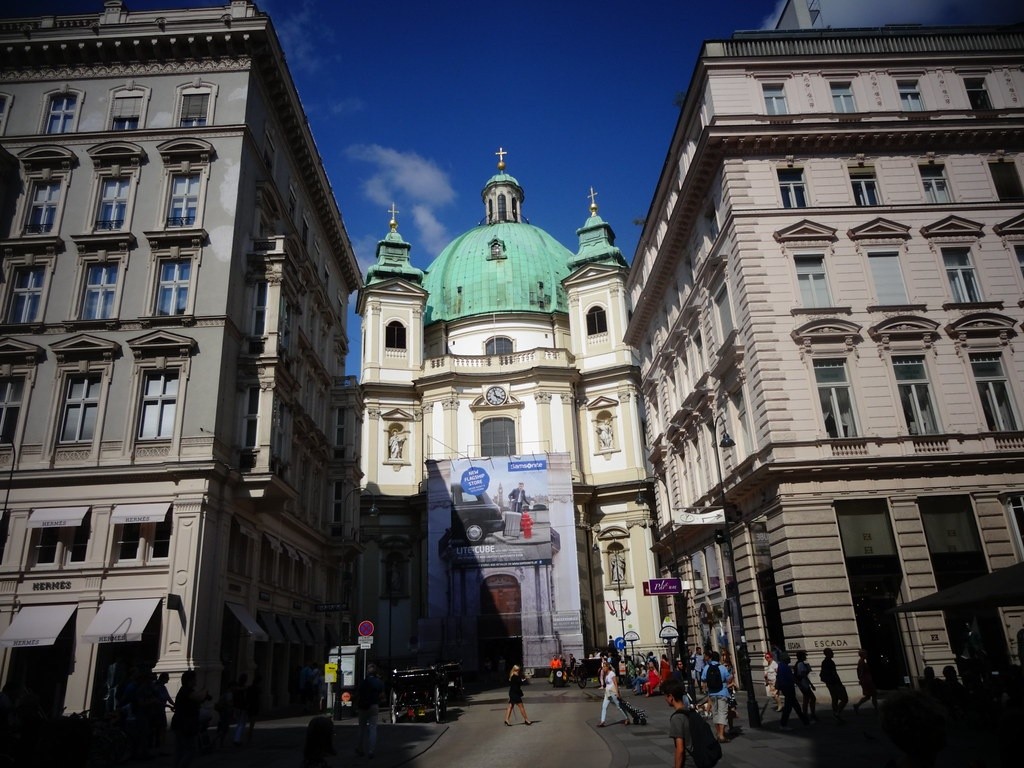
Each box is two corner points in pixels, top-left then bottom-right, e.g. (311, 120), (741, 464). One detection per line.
(858, 649), (869, 659)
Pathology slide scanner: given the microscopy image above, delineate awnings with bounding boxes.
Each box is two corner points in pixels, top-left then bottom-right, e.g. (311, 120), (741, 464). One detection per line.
(225, 602), (339, 647)
(230, 513), (321, 572)
(0, 603), (78, 648)
(25, 506), (91, 527)
(109, 502), (172, 524)
(83, 595), (162, 643)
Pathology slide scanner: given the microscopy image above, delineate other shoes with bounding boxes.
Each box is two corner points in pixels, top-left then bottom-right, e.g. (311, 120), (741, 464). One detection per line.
(597, 722), (607, 726)
(354, 748), (363, 756)
(625, 718), (630, 725)
(832, 712), (841, 721)
(806, 720), (814, 727)
(368, 752), (376, 759)
(809, 713), (821, 721)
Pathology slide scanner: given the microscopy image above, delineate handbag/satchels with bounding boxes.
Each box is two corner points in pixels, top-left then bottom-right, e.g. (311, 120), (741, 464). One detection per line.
(305, 680), (313, 690)
(794, 661), (804, 685)
(775, 669), (795, 692)
(765, 675), (769, 686)
(688, 653), (697, 671)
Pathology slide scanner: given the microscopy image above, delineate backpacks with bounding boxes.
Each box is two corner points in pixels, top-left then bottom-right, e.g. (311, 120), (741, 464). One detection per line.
(670, 709), (723, 768)
(649, 657), (657, 670)
(705, 661), (723, 693)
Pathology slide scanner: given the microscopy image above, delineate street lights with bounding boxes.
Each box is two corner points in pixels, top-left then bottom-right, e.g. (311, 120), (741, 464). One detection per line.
(634, 476), (695, 701)
(714, 410), (763, 729)
(591, 531), (630, 686)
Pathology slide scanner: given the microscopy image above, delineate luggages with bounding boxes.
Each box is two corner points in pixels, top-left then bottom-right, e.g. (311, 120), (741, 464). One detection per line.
(619, 697), (649, 725)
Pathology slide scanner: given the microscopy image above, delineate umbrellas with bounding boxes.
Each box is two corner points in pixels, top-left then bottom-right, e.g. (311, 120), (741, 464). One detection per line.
(884, 561), (1024, 614)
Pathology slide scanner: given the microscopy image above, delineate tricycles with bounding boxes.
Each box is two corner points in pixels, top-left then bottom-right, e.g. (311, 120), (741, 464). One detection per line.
(389, 665), (447, 724)
(432, 657), (463, 696)
(577, 659), (606, 689)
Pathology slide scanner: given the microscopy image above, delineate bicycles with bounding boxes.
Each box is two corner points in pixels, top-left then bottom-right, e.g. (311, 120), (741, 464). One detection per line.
(55, 707), (127, 766)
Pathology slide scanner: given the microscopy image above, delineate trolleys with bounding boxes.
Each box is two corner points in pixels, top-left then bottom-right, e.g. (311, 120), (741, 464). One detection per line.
(685, 692), (715, 721)
(618, 697), (648, 726)
(173, 708), (215, 751)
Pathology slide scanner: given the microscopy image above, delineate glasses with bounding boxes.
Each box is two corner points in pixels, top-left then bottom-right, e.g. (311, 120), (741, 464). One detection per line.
(765, 657), (771, 658)
(514, 670), (519, 672)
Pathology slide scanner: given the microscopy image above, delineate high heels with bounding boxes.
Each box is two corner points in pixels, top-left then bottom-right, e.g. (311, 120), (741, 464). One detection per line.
(505, 721), (511, 726)
(779, 726), (795, 731)
(525, 720), (532, 724)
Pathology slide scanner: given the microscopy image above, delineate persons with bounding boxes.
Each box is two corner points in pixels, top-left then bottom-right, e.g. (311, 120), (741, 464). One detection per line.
(293, 659), (327, 714)
(589, 647), (742, 743)
(116, 669), (269, 767)
(504, 664), (534, 725)
(763, 653), (785, 712)
(793, 650), (820, 723)
(659, 678), (699, 768)
(352, 666), (388, 759)
(548, 654), (580, 688)
(596, 662), (631, 728)
(301, 717), (338, 768)
(820, 648), (849, 722)
(881, 666), (1024, 768)
(777, 651), (817, 731)
(486, 656), (506, 688)
(852, 650), (881, 716)
